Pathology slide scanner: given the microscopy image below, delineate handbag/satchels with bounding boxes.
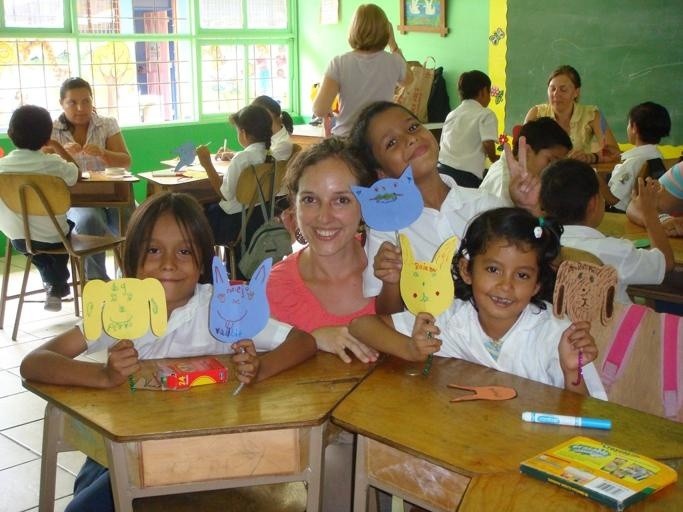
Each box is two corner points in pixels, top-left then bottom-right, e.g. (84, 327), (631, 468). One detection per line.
(427, 66), (450, 123)
(309, 83), (338, 126)
(394, 56), (436, 123)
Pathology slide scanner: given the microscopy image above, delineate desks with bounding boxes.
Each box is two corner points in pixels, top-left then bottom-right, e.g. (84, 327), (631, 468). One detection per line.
(597, 211), (683, 313)
(136, 168), (225, 206)
(22, 347), (387, 512)
(71, 167), (140, 236)
(327, 353), (683, 512)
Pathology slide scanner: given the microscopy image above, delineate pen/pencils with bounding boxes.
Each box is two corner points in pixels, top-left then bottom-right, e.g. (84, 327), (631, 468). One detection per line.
(223, 138), (227, 152)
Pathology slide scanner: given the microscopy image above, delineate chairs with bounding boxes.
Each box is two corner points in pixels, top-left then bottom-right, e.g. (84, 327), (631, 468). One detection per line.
(557, 245), (604, 268)
(221, 160), (291, 282)
(286, 143), (302, 170)
(0, 171), (127, 341)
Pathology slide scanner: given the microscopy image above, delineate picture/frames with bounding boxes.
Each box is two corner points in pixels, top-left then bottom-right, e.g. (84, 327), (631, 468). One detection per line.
(397, 0), (448, 38)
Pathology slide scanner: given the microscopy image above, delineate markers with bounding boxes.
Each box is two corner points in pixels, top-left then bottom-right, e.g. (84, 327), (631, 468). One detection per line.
(522, 411), (612, 430)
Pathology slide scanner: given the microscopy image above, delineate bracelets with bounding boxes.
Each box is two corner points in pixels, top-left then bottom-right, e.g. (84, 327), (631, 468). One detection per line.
(656, 214), (672, 225)
(592, 152), (599, 164)
(391, 46), (398, 52)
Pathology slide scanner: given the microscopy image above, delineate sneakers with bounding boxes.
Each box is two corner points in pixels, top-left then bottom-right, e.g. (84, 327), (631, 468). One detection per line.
(60, 294), (75, 302)
(44, 295), (61, 312)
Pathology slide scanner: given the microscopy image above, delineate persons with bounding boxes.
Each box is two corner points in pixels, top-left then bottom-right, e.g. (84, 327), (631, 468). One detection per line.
(0, 104), (82, 313)
(536, 158), (676, 306)
(19, 190), (317, 512)
(311, 3), (415, 138)
(349, 100), (543, 321)
(262, 137), (382, 364)
(347, 206), (609, 403)
(47, 76), (131, 278)
(251, 95), (295, 217)
(196, 106), (274, 284)
(479, 117), (574, 208)
(522, 64), (622, 165)
(624, 160), (683, 241)
(437, 70), (502, 188)
(594, 101), (671, 213)
